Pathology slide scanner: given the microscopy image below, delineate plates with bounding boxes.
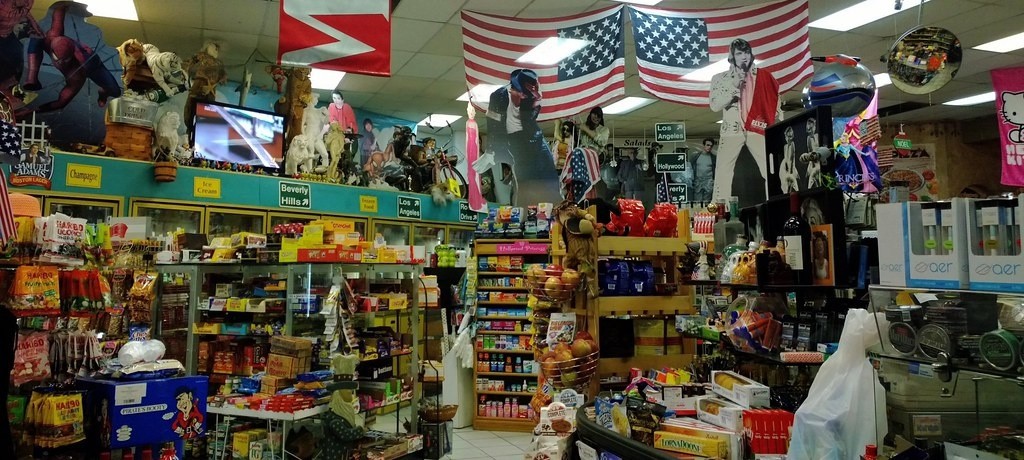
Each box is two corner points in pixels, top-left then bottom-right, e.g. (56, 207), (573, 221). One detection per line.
(882, 170), (924, 194)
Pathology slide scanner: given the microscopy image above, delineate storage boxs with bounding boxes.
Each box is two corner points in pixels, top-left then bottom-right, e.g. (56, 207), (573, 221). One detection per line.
(69, 375), (208, 452)
(873, 195), (1023, 296)
(649, 368), (792, 459)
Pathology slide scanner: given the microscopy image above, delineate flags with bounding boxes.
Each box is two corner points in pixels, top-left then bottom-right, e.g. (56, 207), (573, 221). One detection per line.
(628, 1), (816, 107)
(275, 0), (394, 78)
(460, 4), (628, 122)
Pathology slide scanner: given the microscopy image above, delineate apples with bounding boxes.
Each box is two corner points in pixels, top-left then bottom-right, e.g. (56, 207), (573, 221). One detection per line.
(542, 329), (599, 388)
(526, 263), (580, 298)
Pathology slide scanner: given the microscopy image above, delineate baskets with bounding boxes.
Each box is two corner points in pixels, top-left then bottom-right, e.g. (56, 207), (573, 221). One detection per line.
(418, 405), (458, 422)
(536, 351), (600, 392)
(523, 266), (583, 304)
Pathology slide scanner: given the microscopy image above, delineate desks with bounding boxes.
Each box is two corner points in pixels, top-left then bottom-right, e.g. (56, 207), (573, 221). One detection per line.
(205, 401), (331, 459)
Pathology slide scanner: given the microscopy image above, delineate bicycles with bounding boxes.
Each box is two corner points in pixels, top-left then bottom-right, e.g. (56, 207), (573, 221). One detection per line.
(437, 121), (467, 200)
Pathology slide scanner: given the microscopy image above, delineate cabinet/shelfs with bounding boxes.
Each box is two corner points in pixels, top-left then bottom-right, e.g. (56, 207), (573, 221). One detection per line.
(866, 282), (1024, 460)
(0, 241), (420, 460)
(469, 237), (882, 435)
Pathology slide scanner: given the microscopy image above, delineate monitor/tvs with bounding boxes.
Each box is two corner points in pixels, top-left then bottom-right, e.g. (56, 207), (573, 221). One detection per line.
(187, 98), (288, 174)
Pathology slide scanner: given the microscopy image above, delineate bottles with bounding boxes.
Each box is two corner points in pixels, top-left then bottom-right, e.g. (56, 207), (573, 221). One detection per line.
(498, 353), (504, 371)
(515, 357), (522, 372)
(505, 356), (512, 372)
(483, 353), (490, 372)
(479, 395), (518, 418)
(784, 190), (813, 285)
(491, 354), (497, 372)
(477, 352), (483, 372)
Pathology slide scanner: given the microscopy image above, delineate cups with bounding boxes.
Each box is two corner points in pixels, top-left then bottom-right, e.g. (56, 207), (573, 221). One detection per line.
(119, 340), (165, 366)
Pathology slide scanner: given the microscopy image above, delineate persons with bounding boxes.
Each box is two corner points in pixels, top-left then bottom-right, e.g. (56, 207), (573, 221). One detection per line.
(554, 106), (611, 155)
(184, 42), (226, 150)
(328, 92), (380, 168)
(302, 92), (329, 171)
(695, 248), (707, 266)
(324, 120), (346, 175)
(815, 237), (830, 280)
(15, 3), (121, 116)
(391, 126), (438, 193)
(471, 68), (563, 207)
(800, 199), (825, 226)
(603, 137), (717, 198)
(709, 38), (782, 206)
(778, 119), (821, 195)
(912, 42), (947, 85)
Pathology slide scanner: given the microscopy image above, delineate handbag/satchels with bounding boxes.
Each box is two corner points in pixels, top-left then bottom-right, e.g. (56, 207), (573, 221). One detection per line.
(782, 309), (887, 460)
